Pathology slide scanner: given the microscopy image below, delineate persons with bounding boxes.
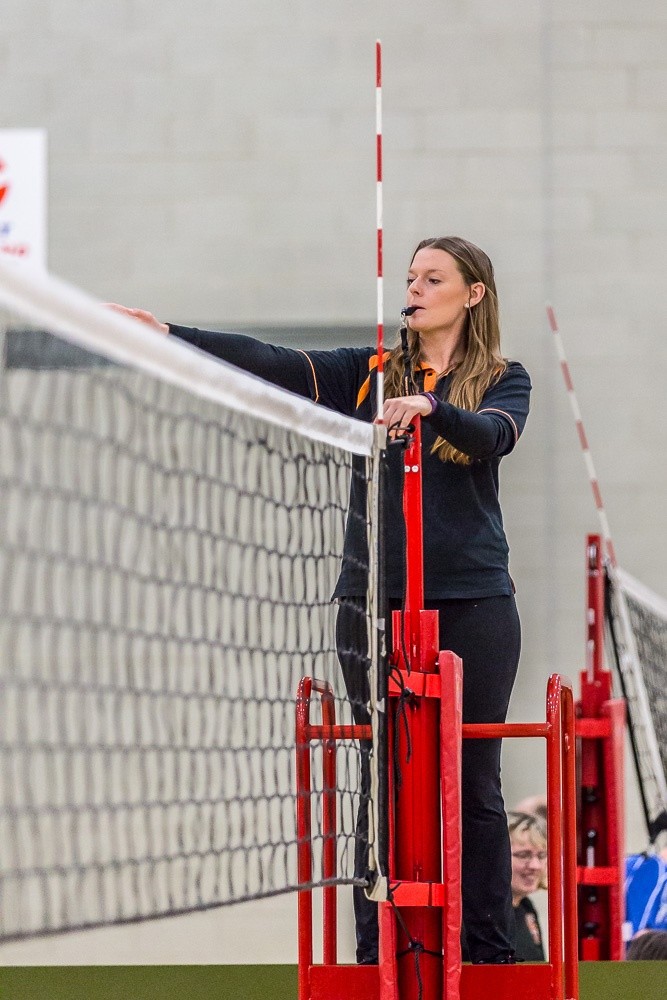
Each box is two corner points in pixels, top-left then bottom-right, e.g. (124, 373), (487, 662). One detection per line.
(505, 812), (667, 962)
(100, 235), (531, 964)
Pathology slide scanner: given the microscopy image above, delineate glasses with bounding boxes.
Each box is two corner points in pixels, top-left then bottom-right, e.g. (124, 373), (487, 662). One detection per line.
(511, 850), (547, 863)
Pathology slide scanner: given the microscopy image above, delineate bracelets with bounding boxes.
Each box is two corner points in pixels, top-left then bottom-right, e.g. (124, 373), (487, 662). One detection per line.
(420, 392), (436, 417)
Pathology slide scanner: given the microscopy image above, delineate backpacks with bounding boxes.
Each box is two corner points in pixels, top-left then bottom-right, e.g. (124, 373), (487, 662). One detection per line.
(623, 851), (667, 941)
